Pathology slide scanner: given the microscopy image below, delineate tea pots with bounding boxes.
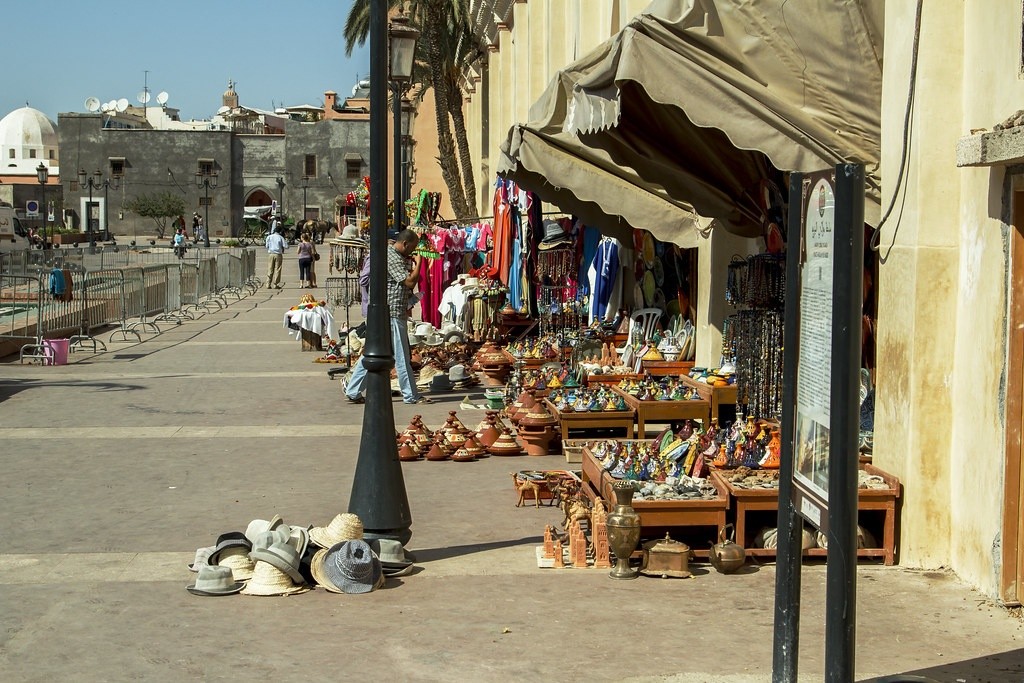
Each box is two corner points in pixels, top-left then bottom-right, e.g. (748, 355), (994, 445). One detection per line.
(706, 523), (747, 573)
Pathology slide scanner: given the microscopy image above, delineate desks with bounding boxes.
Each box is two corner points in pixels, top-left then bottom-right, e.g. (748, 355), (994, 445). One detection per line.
(498, 332), (901, 566)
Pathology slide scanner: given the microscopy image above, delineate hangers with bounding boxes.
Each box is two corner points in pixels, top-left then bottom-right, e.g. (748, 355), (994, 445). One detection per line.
(545, 212), (558, 221)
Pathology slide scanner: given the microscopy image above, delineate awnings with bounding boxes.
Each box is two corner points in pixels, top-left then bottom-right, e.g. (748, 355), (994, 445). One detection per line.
(496, 0), (886, 253)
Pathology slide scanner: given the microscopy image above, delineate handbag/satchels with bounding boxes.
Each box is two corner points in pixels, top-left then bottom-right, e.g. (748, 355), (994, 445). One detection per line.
(315, 254), (320, 261)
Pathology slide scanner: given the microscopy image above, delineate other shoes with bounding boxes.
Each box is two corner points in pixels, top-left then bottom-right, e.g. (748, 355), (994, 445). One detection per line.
(267, 286), (272, 289)
(274, 285), (277, 289)
(296, 285), (303, 288)
(305, 285), (317, 288)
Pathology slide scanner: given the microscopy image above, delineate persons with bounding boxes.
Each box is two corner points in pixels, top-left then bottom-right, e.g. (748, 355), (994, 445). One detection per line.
(171, 212), (319, 289)
(339, 228), (435, 405)
(25, 224), (45, 249)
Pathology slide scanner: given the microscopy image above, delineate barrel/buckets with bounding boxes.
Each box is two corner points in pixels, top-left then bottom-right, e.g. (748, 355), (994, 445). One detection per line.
(43, 338), (70, 365)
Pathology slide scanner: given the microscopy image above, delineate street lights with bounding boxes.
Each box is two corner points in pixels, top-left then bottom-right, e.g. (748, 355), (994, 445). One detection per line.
(299, 175), (310, 220)
(77, 167), (120, 247)
(387, 13), (418, 229)
(36, 161), (49, 261)
(195, 169), (217, 247)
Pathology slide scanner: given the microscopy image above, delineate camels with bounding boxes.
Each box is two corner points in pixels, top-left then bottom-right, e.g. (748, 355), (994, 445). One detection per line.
(509, 472), (593, 545)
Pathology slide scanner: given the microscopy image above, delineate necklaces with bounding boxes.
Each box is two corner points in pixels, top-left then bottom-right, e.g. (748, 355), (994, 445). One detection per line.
(535, 250), (575, 341)
(732, 259), (784, 418)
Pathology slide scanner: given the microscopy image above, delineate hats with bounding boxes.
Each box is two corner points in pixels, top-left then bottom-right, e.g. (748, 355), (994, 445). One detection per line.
(538, 223), (572, 250)
(415, 364), (481, 392)
(451, 274), (485, 290)
(184, 513), (413, 596)
(338, 317), (467, 358)
(387, 229), (400, 239)
(333, 223), (365, 244)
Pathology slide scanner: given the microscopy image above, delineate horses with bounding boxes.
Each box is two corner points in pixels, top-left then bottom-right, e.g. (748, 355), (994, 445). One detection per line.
(295, 219), (339, 245)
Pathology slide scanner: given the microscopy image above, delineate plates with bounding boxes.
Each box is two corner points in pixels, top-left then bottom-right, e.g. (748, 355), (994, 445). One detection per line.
(674, 313), (696, 362)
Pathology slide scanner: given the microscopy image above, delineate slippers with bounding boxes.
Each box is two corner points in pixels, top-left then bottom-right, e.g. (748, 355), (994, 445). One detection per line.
(343, 396), (365, 404)
(406, 395), (433, 404)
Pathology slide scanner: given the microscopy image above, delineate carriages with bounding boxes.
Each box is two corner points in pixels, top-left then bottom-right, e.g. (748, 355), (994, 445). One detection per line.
(236, 205), (332, 247)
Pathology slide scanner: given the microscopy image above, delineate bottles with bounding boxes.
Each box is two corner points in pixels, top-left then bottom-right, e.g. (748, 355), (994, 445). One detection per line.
(478, 337), (786, 501)
